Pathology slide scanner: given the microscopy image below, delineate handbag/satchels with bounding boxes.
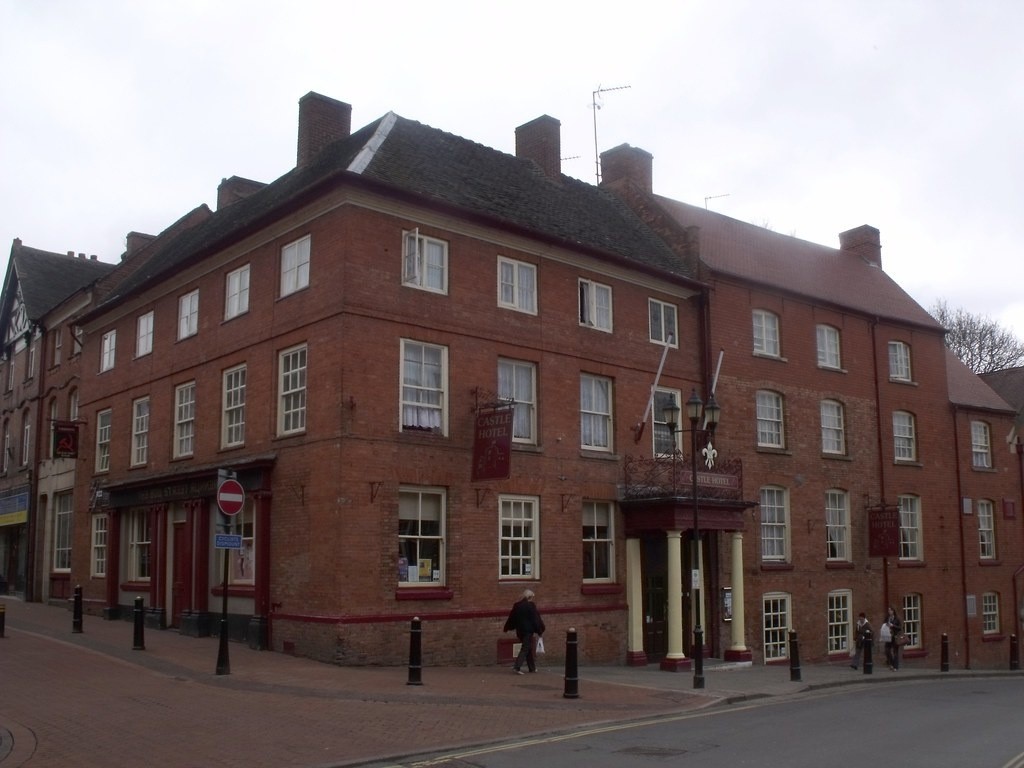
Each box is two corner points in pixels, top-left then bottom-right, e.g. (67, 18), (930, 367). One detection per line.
(894, 629), (910, 645)
(879, 623), (891, 642)
(535, 637), (545, 656)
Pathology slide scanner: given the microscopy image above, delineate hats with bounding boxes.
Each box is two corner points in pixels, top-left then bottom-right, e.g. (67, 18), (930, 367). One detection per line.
(522, 589), (534, 598)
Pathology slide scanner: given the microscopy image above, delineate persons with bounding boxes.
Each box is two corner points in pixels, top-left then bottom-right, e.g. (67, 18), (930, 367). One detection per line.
(883, 607), (901, 672)
(848, 613), (874, 670)
(503, 590), (545, 674)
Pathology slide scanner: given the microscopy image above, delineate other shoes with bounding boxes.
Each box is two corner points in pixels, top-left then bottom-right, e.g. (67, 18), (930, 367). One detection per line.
(528, 668), (537, 672)
(511, 668), (524, 674)
(849, 665), (856, 670)
